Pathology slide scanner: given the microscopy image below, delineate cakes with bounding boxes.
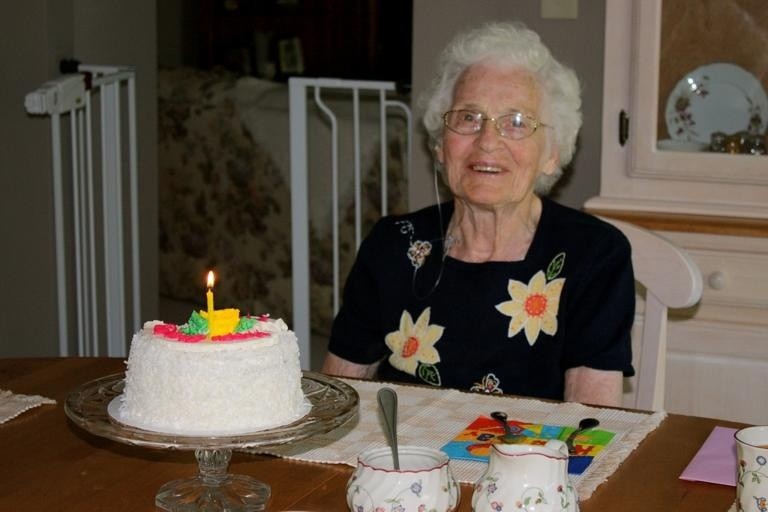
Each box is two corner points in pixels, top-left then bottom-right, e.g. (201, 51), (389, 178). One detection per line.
(121, 308), (308, 434)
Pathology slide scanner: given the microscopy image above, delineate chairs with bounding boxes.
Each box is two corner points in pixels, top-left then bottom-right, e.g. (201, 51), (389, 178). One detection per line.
(594, 213), (705, 415)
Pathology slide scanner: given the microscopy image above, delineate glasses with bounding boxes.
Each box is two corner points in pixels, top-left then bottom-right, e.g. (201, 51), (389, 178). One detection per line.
(443, 109), (553, 140)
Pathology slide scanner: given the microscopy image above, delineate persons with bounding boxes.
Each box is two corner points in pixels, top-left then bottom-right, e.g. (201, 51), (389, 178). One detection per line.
(321, 20), (635, 407)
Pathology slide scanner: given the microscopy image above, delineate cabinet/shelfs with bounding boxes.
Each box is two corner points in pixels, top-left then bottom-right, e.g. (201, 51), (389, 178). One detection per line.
(583, 193), (767, 426)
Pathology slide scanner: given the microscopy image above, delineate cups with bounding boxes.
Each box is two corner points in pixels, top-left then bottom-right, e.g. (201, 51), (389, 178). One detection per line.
(345, 445), (462, 512)
(470, 440), (580, 512)
(729, 424), (767, 512)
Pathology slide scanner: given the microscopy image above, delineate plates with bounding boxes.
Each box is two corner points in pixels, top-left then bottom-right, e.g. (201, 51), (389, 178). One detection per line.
(664, 62), (767, 148)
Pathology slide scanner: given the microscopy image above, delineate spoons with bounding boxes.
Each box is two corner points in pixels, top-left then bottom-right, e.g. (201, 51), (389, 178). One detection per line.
(565, 418), (600, 446)
(491, 411), (511, 431)
